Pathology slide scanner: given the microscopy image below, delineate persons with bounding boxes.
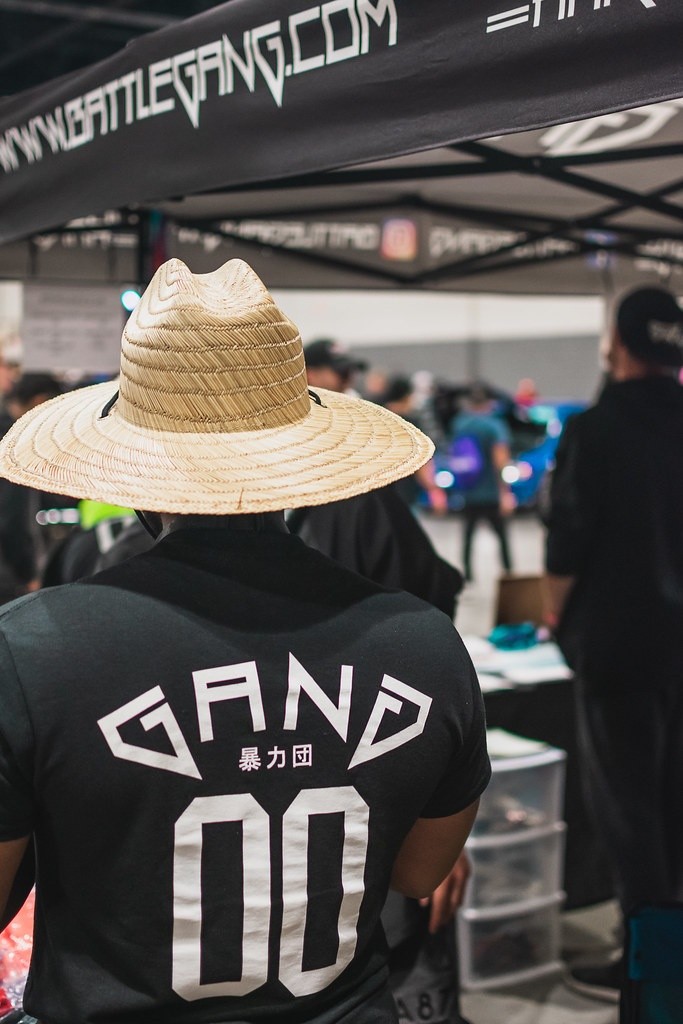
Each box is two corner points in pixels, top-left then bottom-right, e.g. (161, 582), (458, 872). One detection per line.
(283, 338), (465, 623)
(0, 322), (137, 608)
(0, 259), (492, 1023)
(373, 378), (449, 518)
(544, 285), (682, 1024)
(447, 384), (514, 582)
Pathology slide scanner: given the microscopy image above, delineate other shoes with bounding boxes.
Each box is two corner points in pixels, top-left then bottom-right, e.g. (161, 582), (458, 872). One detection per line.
(565, 955), (626, 1004)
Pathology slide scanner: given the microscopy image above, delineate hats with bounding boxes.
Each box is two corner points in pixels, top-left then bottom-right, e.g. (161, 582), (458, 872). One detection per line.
(617, 287), (683, 367)
(1, 257), (434, 517)
(302, 338), (365, 376)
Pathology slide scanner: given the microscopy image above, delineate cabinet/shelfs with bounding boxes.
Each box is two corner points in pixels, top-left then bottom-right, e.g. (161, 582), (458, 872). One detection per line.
(456, 729), (568, 990)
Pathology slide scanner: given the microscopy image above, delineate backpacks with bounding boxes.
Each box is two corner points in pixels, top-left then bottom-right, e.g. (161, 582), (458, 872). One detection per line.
(442, 434), (487, 490)
